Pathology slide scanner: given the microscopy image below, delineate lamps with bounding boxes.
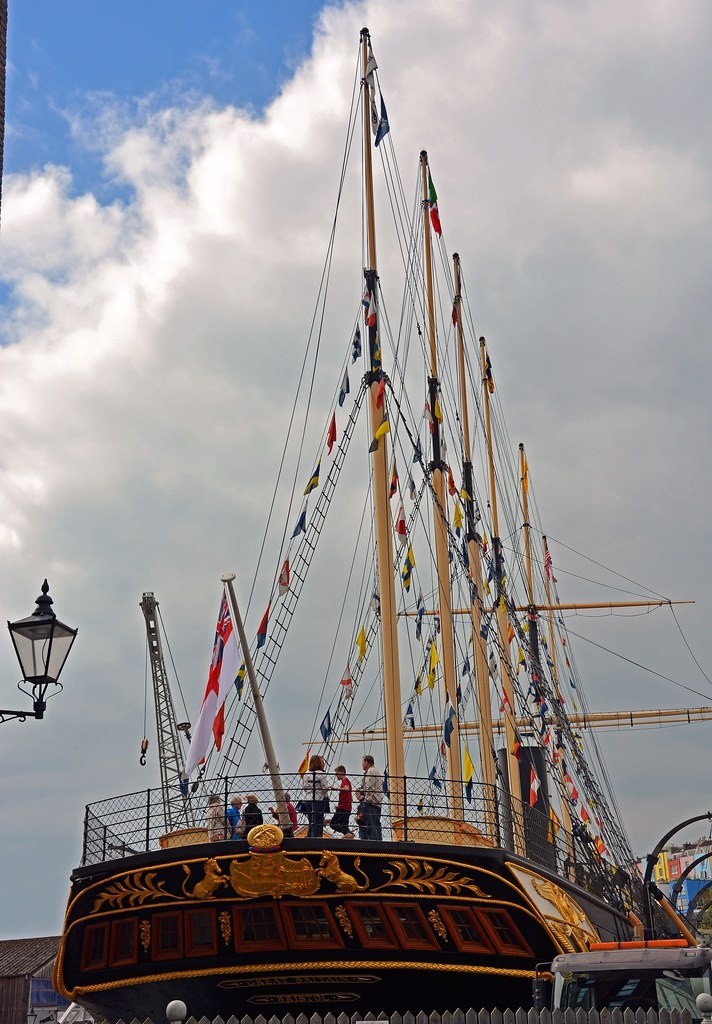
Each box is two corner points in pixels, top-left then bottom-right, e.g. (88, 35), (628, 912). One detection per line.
(26, 1005), (37, 1024)
(0, 578), (78, 726)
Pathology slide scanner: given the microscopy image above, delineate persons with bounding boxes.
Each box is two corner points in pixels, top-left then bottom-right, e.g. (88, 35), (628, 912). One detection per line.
(300, 756), (330, 836)
(328, 765), (355, 838)
(354, 755), (383, 840)
(206, 794), (264, 842)
(268, 793), (297, 837)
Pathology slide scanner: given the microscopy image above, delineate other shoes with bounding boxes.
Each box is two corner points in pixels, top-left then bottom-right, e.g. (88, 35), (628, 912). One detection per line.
(342, 833), (355, 839)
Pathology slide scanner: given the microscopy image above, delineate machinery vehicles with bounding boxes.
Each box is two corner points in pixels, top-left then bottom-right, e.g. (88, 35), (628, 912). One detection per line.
(138, 591), (209, 832)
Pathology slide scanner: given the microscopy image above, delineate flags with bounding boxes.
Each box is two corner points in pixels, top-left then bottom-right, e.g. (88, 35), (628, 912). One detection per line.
(414, 167), (623, 877)
(185, 591), (243, 777)
(195, 29), (414, 772)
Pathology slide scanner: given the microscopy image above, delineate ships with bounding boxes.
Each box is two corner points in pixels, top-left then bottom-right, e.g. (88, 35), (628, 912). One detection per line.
(56, 24), (711, 1022)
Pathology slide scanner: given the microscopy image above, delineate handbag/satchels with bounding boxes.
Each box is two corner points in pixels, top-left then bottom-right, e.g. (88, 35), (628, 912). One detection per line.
(295, 800), (306, 813)
(235, 809), (246, 834)
